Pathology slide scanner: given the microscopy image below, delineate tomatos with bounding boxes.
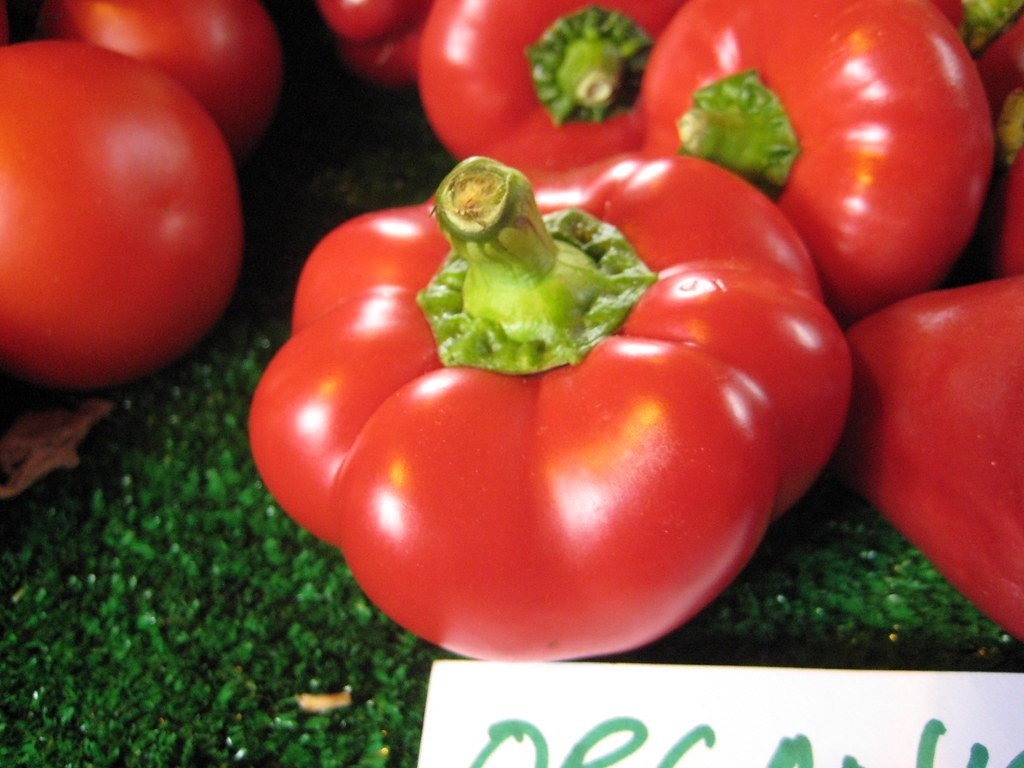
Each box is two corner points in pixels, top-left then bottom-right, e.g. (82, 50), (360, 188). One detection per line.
(0, 1), (286, 390)
(248, 0), (1024, 669)
